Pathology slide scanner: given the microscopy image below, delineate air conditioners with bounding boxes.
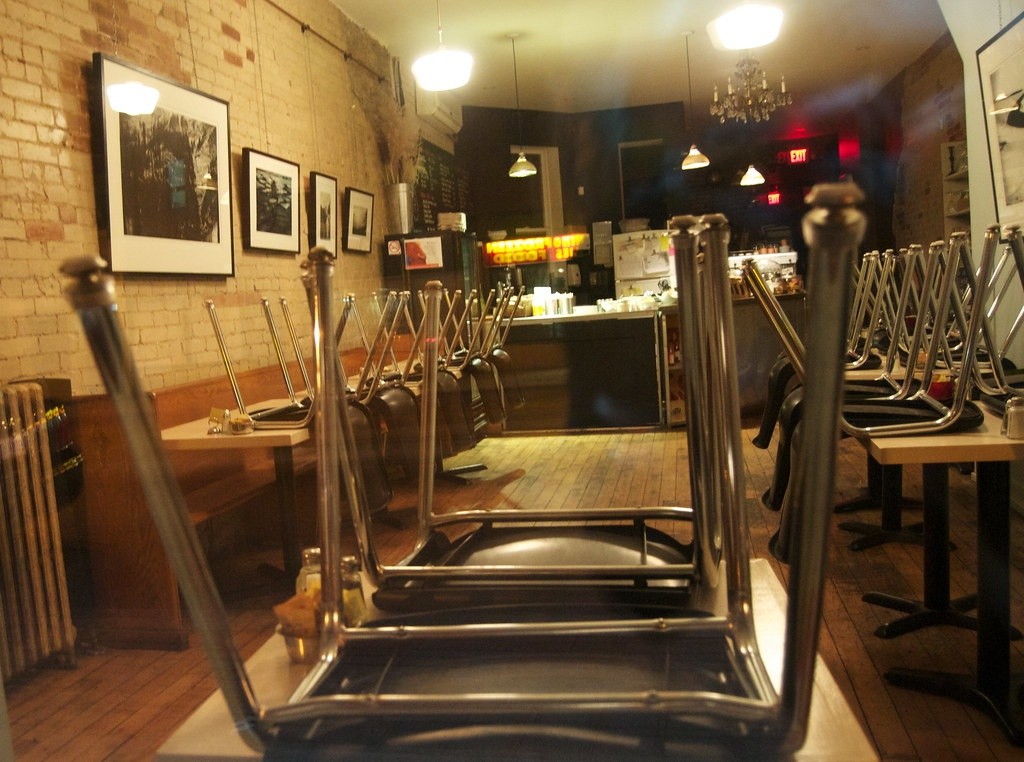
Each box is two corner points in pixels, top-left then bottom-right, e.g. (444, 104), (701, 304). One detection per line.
(416, 85), (463, 136)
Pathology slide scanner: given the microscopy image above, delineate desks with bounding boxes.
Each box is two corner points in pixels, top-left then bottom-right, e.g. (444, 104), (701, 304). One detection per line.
(292, 380), (410, 534)
(151, 558), (878, 762)
(832, 400), (1024, 762)
(380, 356), (488, 479)
(158, 400), (308, 597)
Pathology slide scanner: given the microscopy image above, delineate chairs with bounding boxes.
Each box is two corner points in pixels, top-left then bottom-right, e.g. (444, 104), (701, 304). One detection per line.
(54, 184), (1024, 762)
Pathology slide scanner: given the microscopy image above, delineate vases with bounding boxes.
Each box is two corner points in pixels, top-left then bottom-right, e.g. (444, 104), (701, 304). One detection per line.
(385, 184), (415, 234)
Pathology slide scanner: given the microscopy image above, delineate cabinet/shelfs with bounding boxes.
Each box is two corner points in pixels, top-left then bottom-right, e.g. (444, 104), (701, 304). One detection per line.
(941, 140), (971, 270)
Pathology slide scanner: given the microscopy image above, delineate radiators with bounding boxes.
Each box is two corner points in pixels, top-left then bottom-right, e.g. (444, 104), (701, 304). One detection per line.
(1, 380), (79, 682)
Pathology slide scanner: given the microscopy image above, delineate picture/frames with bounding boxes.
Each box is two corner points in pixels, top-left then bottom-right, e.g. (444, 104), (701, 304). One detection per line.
(976, 10), (1024, 244)
(343, 186), (374, 253)
(92, 52), (236, 277)
(310, 171), (338, 259)
(242, 146), (301, 254)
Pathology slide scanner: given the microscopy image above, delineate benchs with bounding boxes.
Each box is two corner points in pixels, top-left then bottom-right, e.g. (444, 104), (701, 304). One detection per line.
(68, 335), (445, 650)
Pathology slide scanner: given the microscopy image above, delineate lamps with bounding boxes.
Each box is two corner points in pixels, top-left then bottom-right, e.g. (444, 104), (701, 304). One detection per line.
(504, 32), (537, 177)
(739, 166), (764, 187)
(681, 30), (710, 170)
(410, 0), (473, 91)
(705, 4), (793, 125)
